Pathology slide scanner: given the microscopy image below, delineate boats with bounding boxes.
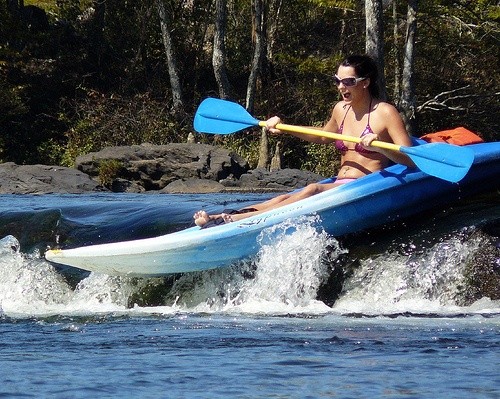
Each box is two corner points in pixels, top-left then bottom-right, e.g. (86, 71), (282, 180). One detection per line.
(44, 142), (500, 276)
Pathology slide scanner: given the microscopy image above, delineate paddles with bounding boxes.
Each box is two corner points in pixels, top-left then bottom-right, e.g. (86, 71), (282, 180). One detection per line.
(191, 97), (477, 185)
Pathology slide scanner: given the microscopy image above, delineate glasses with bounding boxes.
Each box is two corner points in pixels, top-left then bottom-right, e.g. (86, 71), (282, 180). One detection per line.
(334, 74), (367, 87)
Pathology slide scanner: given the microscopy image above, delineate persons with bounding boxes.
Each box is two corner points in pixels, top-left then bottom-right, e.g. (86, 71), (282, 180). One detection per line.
(193, 54), (413, 227)
